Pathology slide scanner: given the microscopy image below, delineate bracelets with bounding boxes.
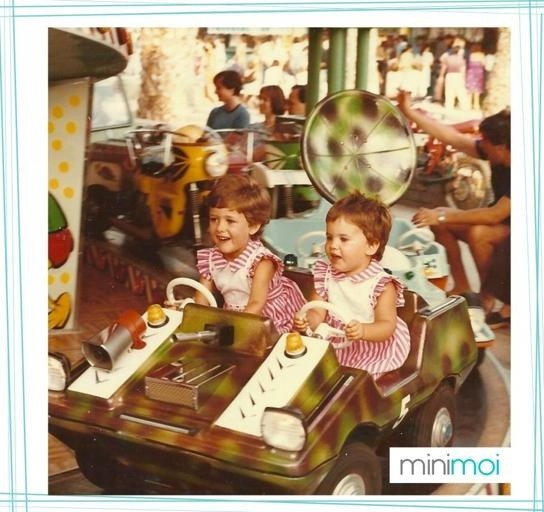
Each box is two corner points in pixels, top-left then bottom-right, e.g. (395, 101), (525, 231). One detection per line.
(434, 207), (448, 228)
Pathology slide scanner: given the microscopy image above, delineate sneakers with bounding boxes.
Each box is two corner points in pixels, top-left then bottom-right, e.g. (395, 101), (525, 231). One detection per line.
(487, 312), (511, 329)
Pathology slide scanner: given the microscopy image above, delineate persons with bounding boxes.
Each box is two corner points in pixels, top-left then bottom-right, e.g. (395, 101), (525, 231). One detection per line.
(200, 70), (250, 150)
(194, 29), (328, 95)
(290, 192), (413, 379)
(478, 234), (509, 330)
(226, 84), (285, 167)
(162, 170), (307, 337)
(284, 83), (307, 119)
(396, 87), (510, 315)
(378, 35), (495, 108)
